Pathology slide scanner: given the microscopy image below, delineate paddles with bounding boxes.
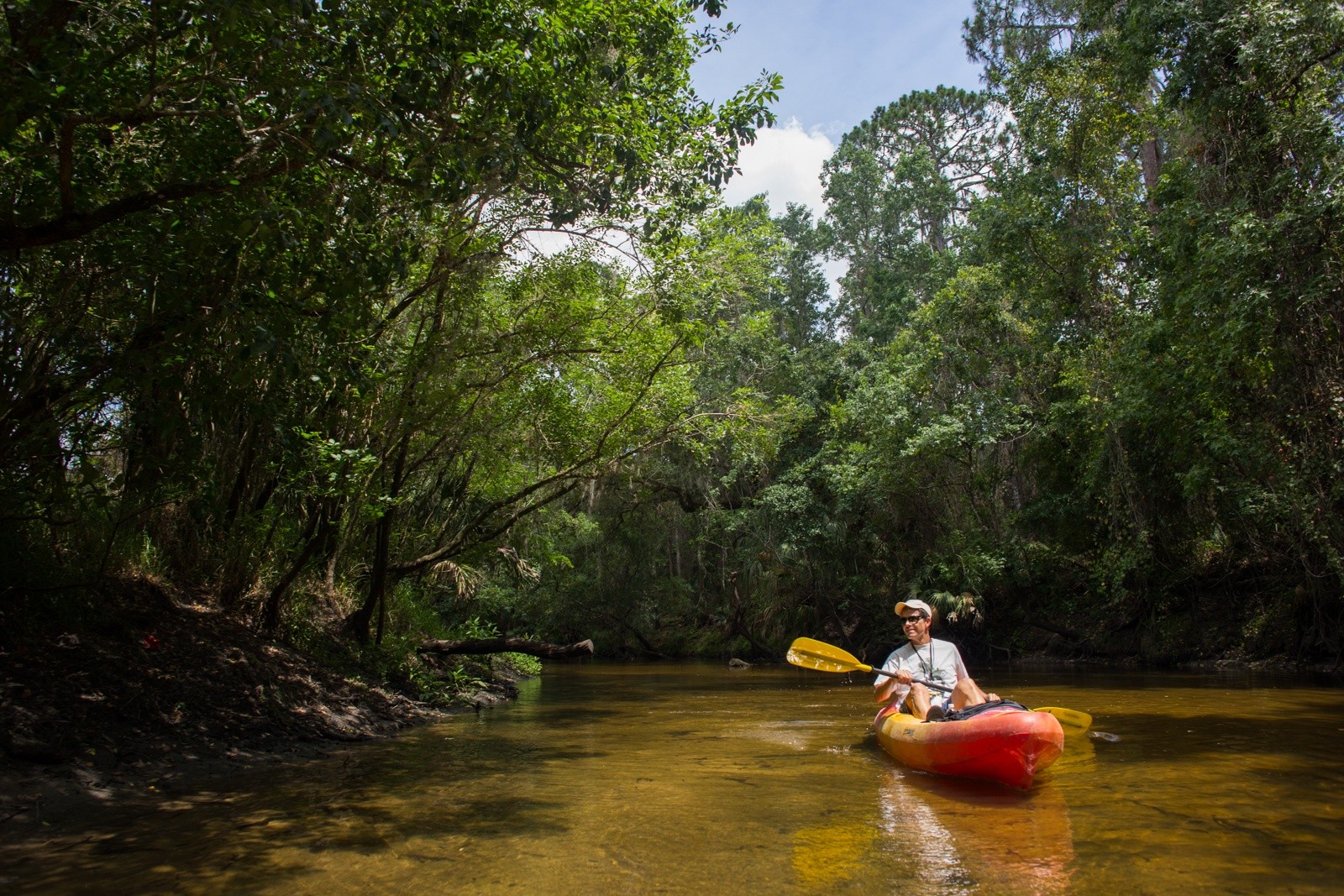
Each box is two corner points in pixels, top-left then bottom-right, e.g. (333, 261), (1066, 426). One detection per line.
(786, 637), (1092, 737)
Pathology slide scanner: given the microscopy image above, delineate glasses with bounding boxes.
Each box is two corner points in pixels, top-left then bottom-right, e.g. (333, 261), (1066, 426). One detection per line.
(901, 616), (927, 624)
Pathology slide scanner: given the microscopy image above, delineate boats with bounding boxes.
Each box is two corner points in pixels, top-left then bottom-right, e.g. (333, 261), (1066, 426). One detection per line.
(873, 697), (1065, 792)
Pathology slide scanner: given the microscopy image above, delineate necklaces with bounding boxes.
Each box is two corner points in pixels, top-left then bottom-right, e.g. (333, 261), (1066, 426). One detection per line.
(911, 639), (935, 680)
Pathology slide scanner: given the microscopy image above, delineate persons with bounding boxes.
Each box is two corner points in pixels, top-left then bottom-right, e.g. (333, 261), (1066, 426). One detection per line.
(873, 598), (1002, 721)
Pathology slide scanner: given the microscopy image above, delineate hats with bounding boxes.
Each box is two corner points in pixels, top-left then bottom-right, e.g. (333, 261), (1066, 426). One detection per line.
(894, 599), (932, 618)
